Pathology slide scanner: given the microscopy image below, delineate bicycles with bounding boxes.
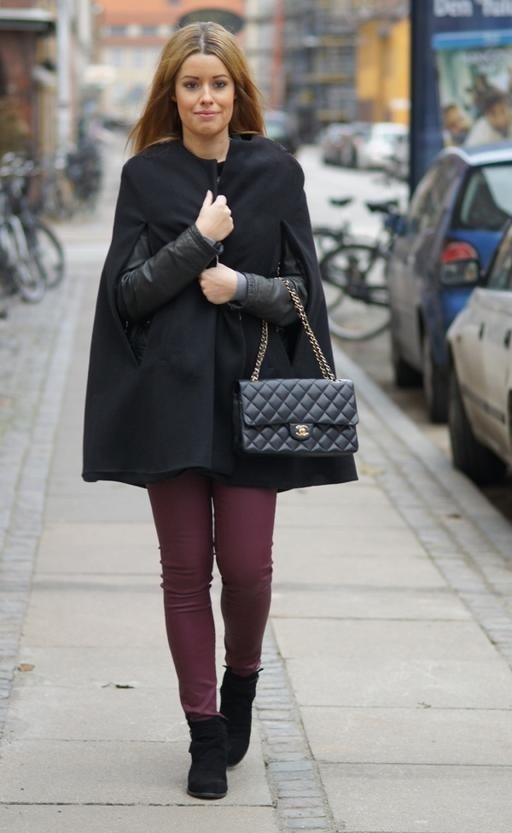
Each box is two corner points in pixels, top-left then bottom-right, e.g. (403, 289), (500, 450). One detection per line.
(1, 149), (65, 305)
(310, 195), (399, 343)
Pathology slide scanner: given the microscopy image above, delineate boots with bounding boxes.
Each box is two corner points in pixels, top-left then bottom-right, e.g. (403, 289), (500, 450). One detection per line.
(215, 665), (261, 773)
(183, 713), (229, 800)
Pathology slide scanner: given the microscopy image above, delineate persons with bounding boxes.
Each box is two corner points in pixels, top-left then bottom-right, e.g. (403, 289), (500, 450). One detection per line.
(437, 104), (470, 146)
(75, 19), (339, 802)
(457, 93), (512, 145)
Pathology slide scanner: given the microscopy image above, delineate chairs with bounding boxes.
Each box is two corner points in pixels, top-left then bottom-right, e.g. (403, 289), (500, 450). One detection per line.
(467, 178), (510, 228)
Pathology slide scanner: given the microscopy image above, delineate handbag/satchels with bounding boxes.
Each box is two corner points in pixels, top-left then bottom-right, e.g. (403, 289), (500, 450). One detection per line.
(228, 277), (361, 462)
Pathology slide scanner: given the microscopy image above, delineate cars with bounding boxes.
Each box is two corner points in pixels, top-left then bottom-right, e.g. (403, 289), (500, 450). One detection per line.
(321, 120), (410, 180)
